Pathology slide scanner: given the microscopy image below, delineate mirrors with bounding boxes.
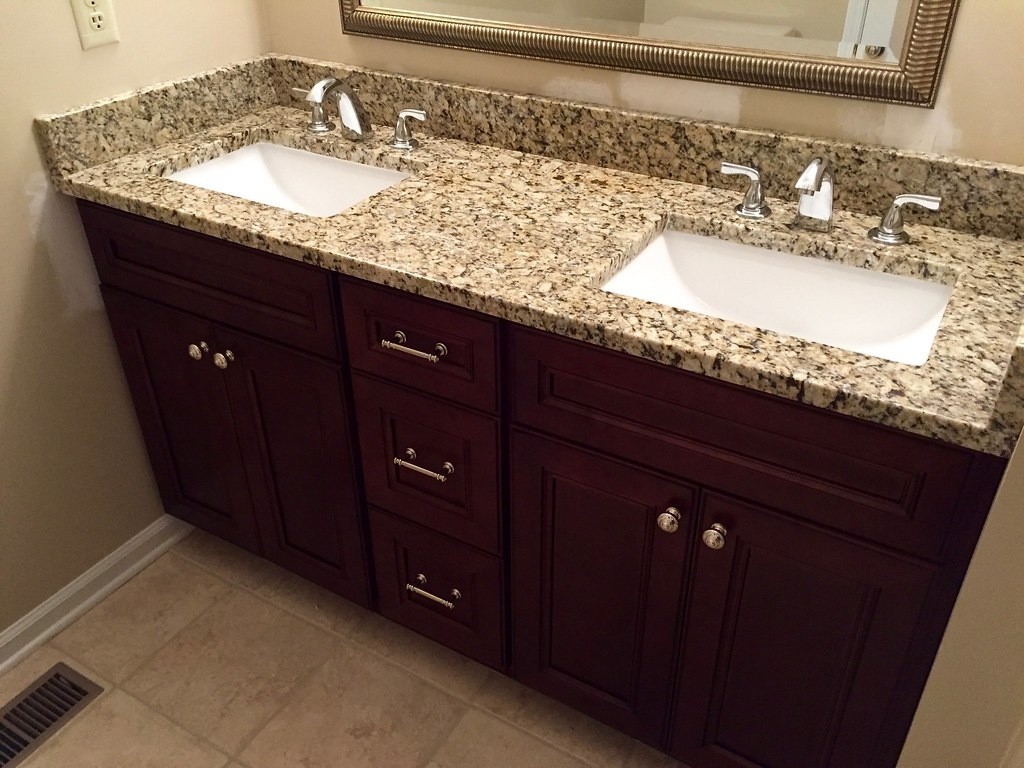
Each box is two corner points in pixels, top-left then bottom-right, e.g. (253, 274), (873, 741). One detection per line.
(340, 0), (959, 110)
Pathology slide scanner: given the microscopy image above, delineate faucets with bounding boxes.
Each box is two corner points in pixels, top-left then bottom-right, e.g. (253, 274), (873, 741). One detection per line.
(794, 154), (834, 232)
(304, 77), (375, 142)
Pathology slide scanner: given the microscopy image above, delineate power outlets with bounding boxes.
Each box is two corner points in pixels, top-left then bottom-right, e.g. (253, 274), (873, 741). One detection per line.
(71, 0), (120, 51)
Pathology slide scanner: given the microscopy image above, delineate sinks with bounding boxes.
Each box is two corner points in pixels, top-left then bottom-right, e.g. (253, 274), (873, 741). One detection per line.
(587, 213), (960, 367)
(148, 124), (429, 218)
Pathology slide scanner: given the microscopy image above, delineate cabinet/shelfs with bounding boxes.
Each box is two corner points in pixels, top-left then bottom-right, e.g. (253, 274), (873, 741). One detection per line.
(76, 199), (1009, 768)
